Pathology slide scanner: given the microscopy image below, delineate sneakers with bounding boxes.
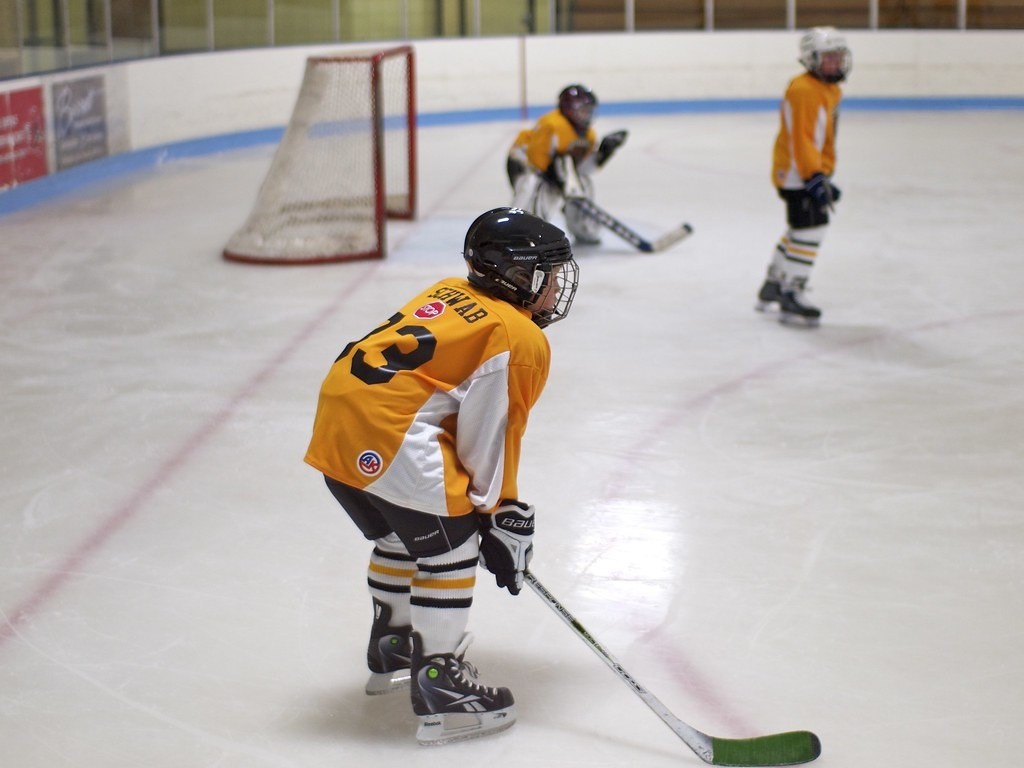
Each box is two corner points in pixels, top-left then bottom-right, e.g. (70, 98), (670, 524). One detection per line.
(366, 595), (414, 695)
(755, 279), (784, 311)
(779, 291), (820, 329)
(410, 631), (517, 746)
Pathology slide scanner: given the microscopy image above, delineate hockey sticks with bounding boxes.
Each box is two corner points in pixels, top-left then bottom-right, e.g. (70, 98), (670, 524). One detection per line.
(535, 167), (693, 254)
(816, 176), (837, 215)
(521, 570), (821, 768)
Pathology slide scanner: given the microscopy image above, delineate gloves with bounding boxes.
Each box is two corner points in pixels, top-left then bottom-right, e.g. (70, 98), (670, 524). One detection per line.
(804, 173), (840, 204)
(478, 499), (535, 596)
(598, 129), (627, 162)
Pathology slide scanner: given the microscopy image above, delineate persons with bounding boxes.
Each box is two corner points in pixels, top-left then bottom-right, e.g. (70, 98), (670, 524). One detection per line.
(508, 84), (628, 245)
(756, 23), (851, 329)
(303, 206), (581, 746)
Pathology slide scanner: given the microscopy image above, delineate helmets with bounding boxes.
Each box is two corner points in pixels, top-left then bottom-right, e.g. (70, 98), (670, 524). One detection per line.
(799, 26), (847, 83)
(559, 86), (595, 136)
(463, 208), (573, 329)
